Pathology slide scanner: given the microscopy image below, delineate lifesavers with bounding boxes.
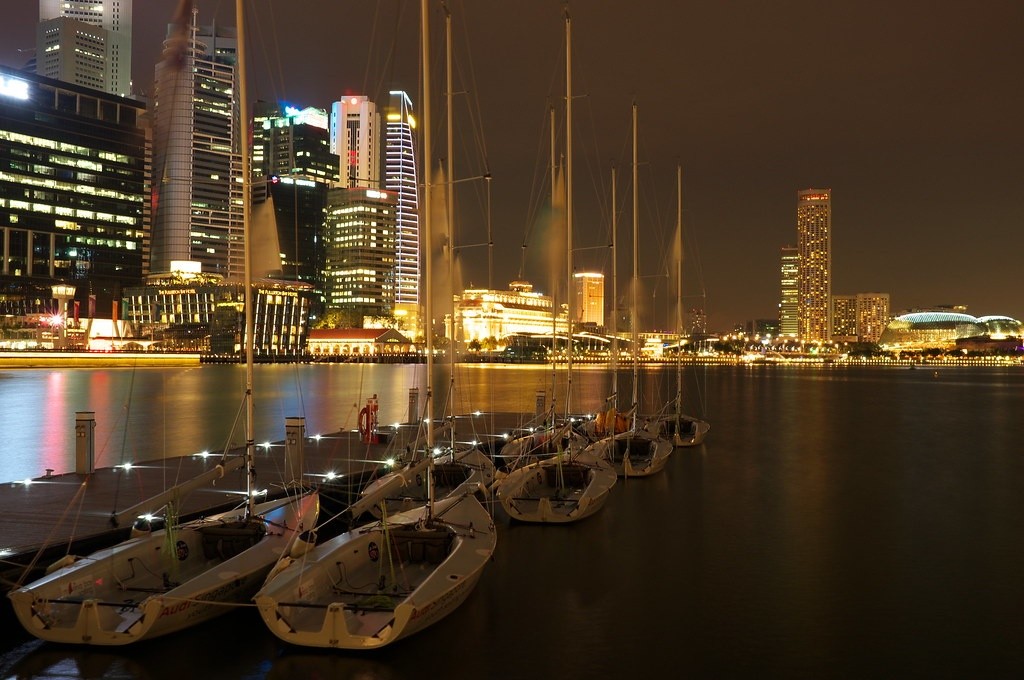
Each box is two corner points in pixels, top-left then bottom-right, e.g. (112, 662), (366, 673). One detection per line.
(359, 406), (374, 435)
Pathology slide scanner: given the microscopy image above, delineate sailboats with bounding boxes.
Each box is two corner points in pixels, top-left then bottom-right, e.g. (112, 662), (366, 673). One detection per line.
(493, 7), (619, 523)
(643, 163), (711, 447)
(5, 0), (321, 645)
(250, 0), (498, 650)
(500, 108), (674, 478)
(360, 0), (495, 521)
